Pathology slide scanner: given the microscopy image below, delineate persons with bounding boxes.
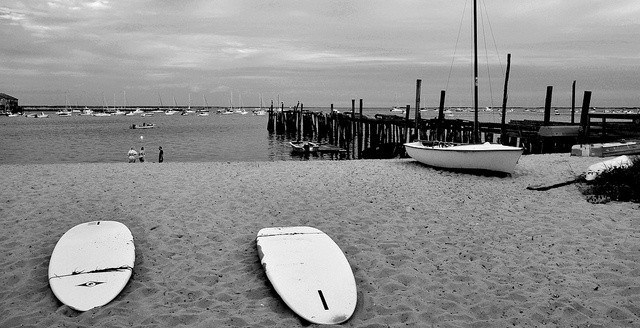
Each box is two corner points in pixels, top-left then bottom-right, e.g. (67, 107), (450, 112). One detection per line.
(127, 147), (138, 163)
(138, 147), (145, 162)
(158, 146), (163, 163)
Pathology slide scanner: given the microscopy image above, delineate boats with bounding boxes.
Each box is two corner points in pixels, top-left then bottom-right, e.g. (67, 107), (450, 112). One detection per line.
(289, 139), (351, 154)
(55, 87), (285, 117)
(390, 107), (404, 112)
(359, 142), (400, 158)
(329, 109), (355, 116)
(129, 121), (157, 129)
(1, 110), (49, 118)
(403, 1), (529, 178)
(532, 107), (639, 115)
(415, 103), (528, 117)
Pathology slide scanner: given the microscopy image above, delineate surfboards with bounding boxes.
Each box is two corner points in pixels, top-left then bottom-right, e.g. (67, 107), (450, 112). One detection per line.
(257, 226), (358, 325)
(48, 220), (135, 312)
